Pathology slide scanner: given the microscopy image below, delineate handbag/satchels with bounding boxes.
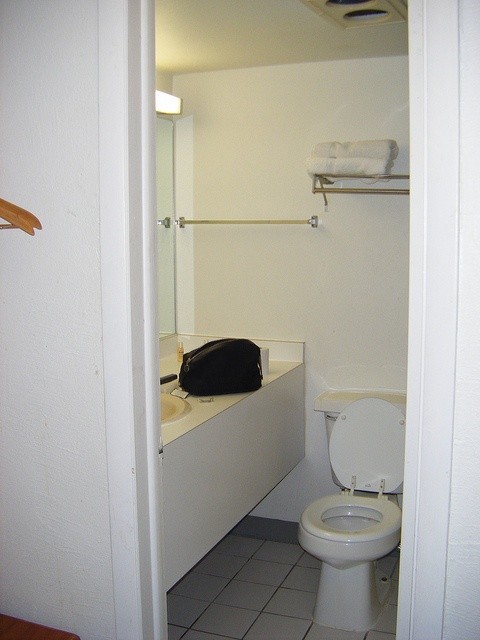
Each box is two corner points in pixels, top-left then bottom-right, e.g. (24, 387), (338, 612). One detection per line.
(179, 337), (264, 397)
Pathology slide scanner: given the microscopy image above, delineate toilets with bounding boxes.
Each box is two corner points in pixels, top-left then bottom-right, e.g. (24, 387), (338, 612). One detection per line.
(295, 389), (408, 634)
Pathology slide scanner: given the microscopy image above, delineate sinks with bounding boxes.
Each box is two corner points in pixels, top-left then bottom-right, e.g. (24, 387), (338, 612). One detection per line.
(160, 391), (186, 423)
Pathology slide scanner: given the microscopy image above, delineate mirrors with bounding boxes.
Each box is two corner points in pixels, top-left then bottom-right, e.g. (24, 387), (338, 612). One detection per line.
(157, 118), (176, 339)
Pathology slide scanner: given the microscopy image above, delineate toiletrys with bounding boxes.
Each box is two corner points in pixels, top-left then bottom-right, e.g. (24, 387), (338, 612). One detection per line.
(176, 342), (184, 364)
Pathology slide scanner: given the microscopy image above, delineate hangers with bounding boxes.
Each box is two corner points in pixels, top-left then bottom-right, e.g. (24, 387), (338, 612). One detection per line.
(1, 199), (43, 237)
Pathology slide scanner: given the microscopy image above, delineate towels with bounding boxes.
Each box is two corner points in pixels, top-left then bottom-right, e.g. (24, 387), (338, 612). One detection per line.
(305, 160), (395, 177)
(308, 139), (400, 158)
